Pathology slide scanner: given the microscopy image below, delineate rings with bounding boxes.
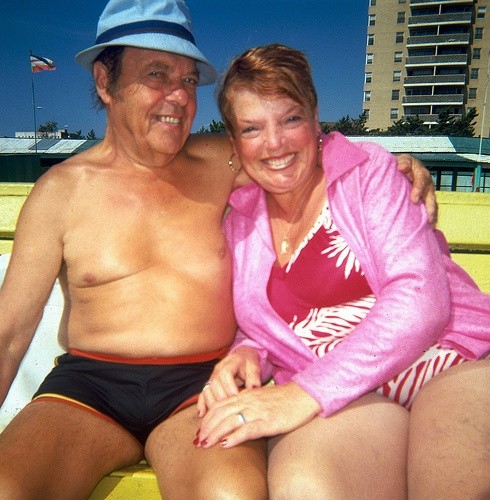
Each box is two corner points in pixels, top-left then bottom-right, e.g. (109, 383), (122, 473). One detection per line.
(239, 413), (245, 424)
(202, 382), (209, 389)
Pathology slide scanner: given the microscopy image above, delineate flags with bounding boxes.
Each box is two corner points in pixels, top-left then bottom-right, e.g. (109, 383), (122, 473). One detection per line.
(30, 55), (56, 72)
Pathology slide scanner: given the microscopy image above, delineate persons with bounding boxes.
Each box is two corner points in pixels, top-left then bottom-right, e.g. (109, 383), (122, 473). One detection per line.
(0, 0), (437, 500)
(194, 43), (490, 500)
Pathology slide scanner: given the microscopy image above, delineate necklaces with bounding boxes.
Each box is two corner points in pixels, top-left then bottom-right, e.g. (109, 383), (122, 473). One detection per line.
(273, 178), (312, 254)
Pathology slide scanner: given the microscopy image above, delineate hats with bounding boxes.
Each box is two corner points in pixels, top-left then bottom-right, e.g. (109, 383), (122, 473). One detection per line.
(74, 0), (218, 87)
